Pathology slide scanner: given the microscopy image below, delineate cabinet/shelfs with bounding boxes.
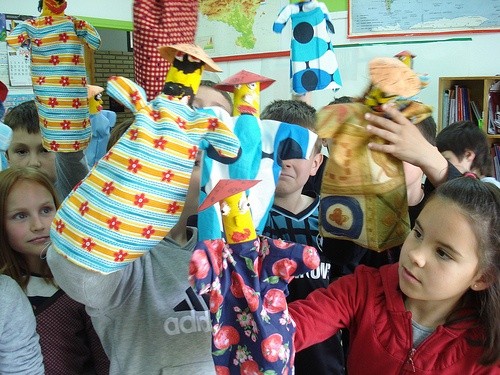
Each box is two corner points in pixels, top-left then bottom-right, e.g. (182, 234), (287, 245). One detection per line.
(437, 76), (500, 179)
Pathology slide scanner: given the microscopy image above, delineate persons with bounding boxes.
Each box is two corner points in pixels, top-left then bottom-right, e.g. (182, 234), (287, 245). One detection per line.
(0, 274), (46, 375)
(357, 101), (464, 269)
(436, 119), (500, 187)
(0, 165), (110, 375)
(260, 100), (347, 375)
(190, 80), (234, 118)
(45, 118), (218, 375)
(285, 171), (500, 375)
(4, 99), (91, 205)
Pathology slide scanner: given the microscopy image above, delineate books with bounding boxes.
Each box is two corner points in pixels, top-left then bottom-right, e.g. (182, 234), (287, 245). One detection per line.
(442, 85), (482, 130)
